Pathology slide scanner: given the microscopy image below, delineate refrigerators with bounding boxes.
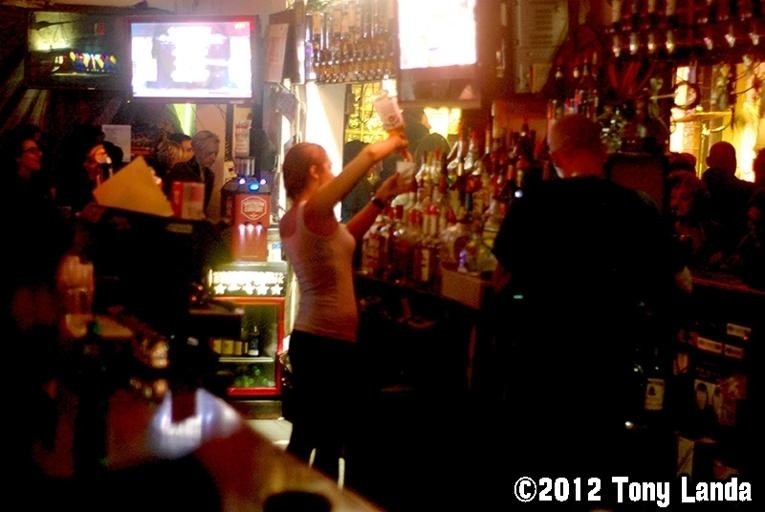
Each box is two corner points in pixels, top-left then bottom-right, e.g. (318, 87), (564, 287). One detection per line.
(199, 262), (294, 420)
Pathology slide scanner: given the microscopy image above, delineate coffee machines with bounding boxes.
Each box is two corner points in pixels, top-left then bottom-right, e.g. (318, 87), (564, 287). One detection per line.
(226, 222), (267, 264)
(220, 155), (271, 229)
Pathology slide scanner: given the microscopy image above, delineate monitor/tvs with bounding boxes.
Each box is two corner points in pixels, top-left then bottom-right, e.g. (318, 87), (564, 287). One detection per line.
(24, 7), (134, 90)
(125, 15), (257, 104)
(392, 1), (498, 107)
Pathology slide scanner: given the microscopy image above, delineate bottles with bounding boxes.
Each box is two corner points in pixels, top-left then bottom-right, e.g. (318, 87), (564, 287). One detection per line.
(625, 308), (668, 441)
(374, 94), (413, 160)
(359, 124), (554, 287)
(606, 0), (765, 57)
(552, 50), (604, 122)
(211, 322), (276, 387)
(310, 21), (399, 84)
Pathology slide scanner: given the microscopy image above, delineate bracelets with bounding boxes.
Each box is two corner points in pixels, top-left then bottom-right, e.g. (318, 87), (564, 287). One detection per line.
(370, 195), (386, 209)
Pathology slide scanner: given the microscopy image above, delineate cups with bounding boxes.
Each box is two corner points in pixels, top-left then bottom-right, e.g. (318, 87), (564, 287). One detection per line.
(170, 180), (206, 211)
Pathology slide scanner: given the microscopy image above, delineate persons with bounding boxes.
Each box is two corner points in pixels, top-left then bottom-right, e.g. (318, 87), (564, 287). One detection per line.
(661, 137), (764, 287)
(344, 109), (450, 218)
(0, 123), (225, 238)
(695, 383), (711, 406)
(490, 113), (664, 512)
(280, 131), (418, 486)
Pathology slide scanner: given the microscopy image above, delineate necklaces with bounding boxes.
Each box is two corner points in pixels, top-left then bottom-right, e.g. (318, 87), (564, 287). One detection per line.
(567, 169), (606, 178)
